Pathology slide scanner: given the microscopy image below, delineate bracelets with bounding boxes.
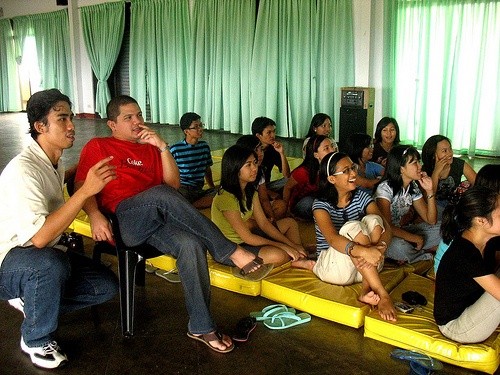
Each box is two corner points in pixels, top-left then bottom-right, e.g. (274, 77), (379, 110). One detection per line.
(345, 241), (359, 258)
(161, 147), (171, 152)
(380, 241), (388, 249)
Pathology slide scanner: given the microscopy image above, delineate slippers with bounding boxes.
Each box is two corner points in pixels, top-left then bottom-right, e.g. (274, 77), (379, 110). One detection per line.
(187, 330), (235, 353)
(155, 267), (182, 282)
(250, 304), (296, 321)
(263, 311), (311, 329)
(145, 260), (157, 273)
(232, 255), (274, 282)
(390, 349), (443, 369)
(410, 360), (438, 375)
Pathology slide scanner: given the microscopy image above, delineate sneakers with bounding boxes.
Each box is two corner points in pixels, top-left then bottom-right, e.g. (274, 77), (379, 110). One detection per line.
(20, 333), (68, 368)
(8, 296), (27, 319)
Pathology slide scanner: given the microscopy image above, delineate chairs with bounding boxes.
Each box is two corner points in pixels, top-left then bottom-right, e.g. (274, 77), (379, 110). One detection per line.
(68, 173), (167, 340)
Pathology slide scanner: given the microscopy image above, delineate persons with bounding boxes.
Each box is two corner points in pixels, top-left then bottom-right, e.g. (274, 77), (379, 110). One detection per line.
(227, 111), (500, 264)
(432, 185), (500, 344)
(311, 152), (402, 323)
(0, 88), (118, 370)
(168, 112), (221, 217)
(209, 144), (315, 271)
(72, 94), (275, 353)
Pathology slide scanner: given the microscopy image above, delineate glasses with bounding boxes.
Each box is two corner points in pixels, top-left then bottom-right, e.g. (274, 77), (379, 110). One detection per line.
(186, 124), (205, 129)
(332, 164), (358, 175)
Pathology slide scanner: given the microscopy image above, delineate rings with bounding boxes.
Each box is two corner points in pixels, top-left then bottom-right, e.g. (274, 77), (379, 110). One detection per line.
(442, 160), (444, 163)
(97, 235), (101, 238)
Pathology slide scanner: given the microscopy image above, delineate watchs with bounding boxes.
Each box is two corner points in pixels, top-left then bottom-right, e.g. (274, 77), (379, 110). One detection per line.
(426, 193), (434, 199)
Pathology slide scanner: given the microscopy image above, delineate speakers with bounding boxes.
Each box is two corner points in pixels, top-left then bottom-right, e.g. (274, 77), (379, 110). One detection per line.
(339, 108), (374, 148)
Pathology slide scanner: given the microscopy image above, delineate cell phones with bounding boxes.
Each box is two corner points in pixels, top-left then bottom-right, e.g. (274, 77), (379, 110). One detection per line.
(393, 301), (413, 314)
